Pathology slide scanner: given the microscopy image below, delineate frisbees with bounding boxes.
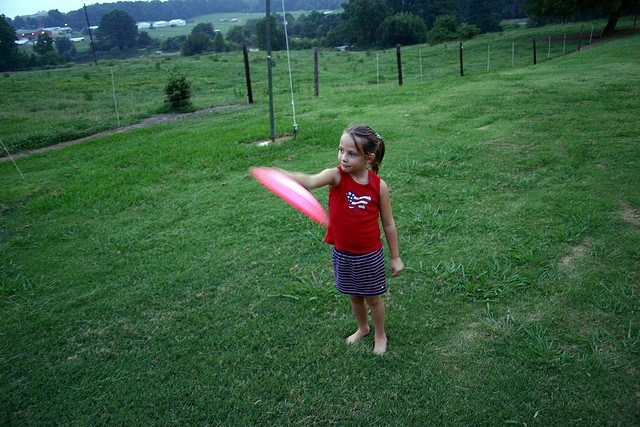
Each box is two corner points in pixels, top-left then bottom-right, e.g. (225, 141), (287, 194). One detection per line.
(250, 166), (330, 227)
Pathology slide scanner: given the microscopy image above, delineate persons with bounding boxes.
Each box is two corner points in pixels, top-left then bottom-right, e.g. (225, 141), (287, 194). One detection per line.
(271, 123), (407, 356)
